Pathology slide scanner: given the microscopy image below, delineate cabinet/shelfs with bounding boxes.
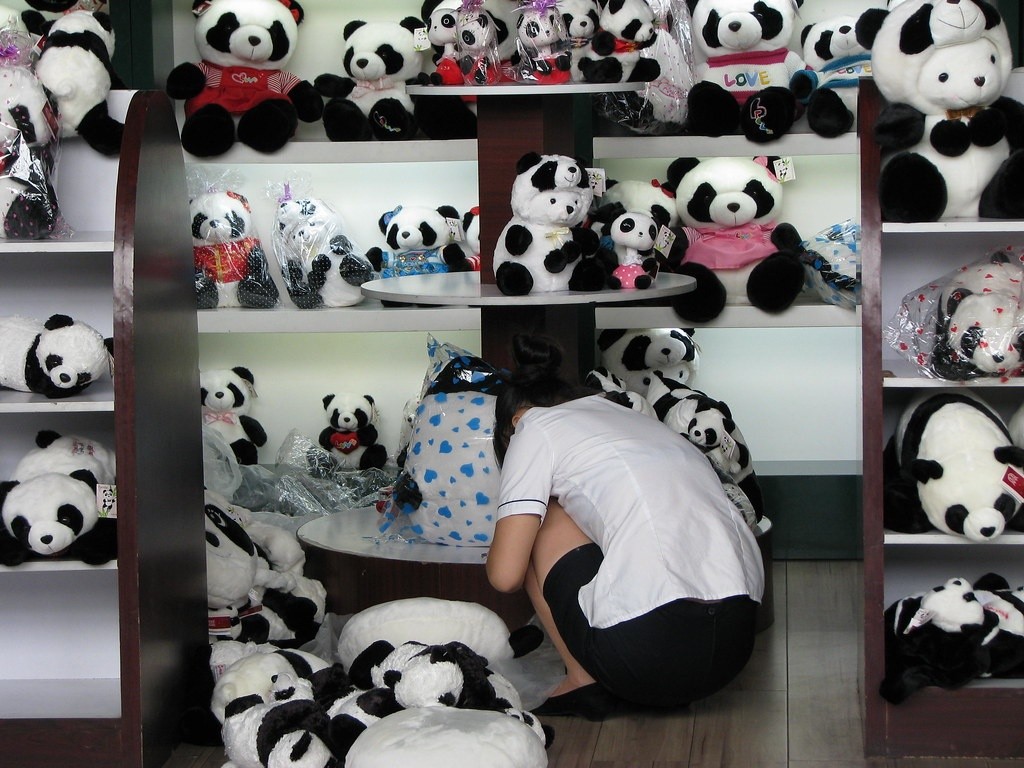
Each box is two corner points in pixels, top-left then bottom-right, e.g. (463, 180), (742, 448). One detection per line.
(1, 1), (1024, 768)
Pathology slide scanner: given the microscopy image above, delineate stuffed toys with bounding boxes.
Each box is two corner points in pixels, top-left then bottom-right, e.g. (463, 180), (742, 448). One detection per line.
(856, 0), (1024, 221)
(447, 206), (480, 273)
(583, 328), (765, 532)
(879, 571), (1024, 705)
(165, 0), (324, 158)
(582, 155), (855, 321)
(931, 252), (1024, 380)
(883, 388), (1024, 541)
(314, 16), (429, 141)
(276, 196), (374, 309)
(0, 0), (127, 244)
(790, 14), (874, 136)
(198, 355), (554, 768)
(493, 151), (610, 294)
(413, 0), (692, 140)
(189, 189), (280, 308)
(685, 0), (812, 143)
(0, 431), (119, 565)
(0, 311), (115, 401)
(365, 203), (466, 308)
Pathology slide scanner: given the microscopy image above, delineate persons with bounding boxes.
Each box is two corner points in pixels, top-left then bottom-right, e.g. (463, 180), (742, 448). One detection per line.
(485, 326), (765, 721)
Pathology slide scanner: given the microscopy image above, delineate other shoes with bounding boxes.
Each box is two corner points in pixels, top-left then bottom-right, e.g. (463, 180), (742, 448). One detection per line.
(520, 672), (611, 724)
